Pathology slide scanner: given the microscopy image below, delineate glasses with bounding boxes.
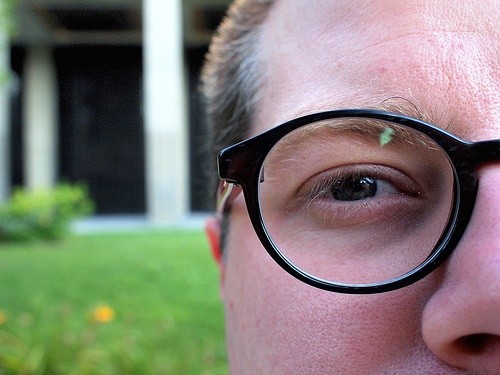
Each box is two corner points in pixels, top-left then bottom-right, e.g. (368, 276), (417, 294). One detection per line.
(217, 109), (500, 294)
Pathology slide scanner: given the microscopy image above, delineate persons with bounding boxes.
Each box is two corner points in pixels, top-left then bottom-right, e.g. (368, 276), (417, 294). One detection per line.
(192, 0), (500, 375)
